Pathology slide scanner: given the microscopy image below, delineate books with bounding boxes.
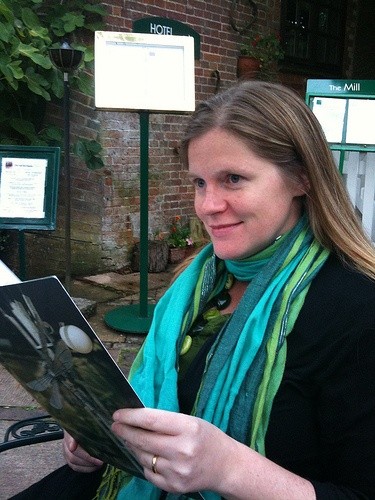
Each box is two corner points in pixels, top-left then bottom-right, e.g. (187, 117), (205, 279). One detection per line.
(0, 259), (206, 500)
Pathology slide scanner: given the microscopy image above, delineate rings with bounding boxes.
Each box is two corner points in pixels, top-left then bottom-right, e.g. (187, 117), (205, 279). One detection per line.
(151, 455), (159, 474)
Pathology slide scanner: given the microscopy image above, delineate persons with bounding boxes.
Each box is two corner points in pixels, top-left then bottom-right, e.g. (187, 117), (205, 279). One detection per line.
(61, 80), (375, 500)
